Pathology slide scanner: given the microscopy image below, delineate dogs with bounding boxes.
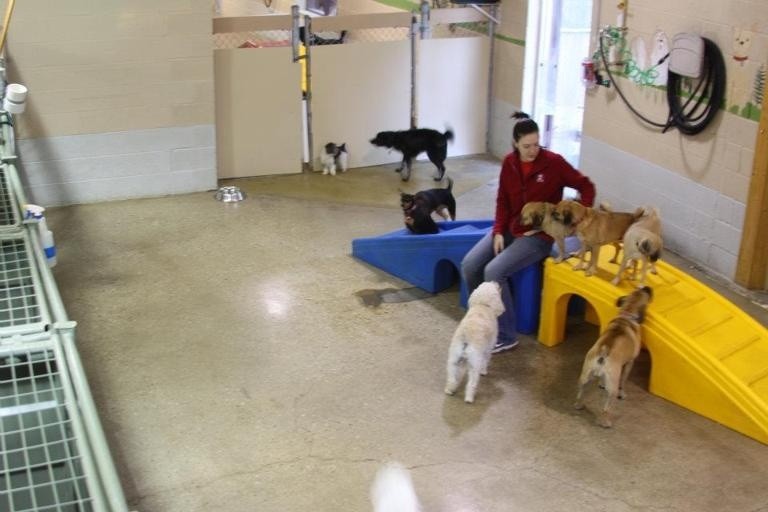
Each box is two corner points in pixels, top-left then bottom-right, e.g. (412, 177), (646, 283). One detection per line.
(370, 128), (454, 182)
(319, 142), (349, 176)
(519, 200), (664, 290)
(444, 280), (506, 404)
(400, 177), (456, 234)
(574, 286), (654, 428)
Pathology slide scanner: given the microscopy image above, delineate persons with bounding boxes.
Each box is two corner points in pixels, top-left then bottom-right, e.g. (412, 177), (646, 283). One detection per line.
(459, 108), (597, 353)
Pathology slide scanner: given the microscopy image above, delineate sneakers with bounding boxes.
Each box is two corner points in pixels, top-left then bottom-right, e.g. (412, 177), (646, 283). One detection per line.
(491, 338), (519, 354)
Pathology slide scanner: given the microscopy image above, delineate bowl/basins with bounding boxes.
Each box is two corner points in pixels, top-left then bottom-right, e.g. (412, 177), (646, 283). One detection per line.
(215, 186), (244, 202)
